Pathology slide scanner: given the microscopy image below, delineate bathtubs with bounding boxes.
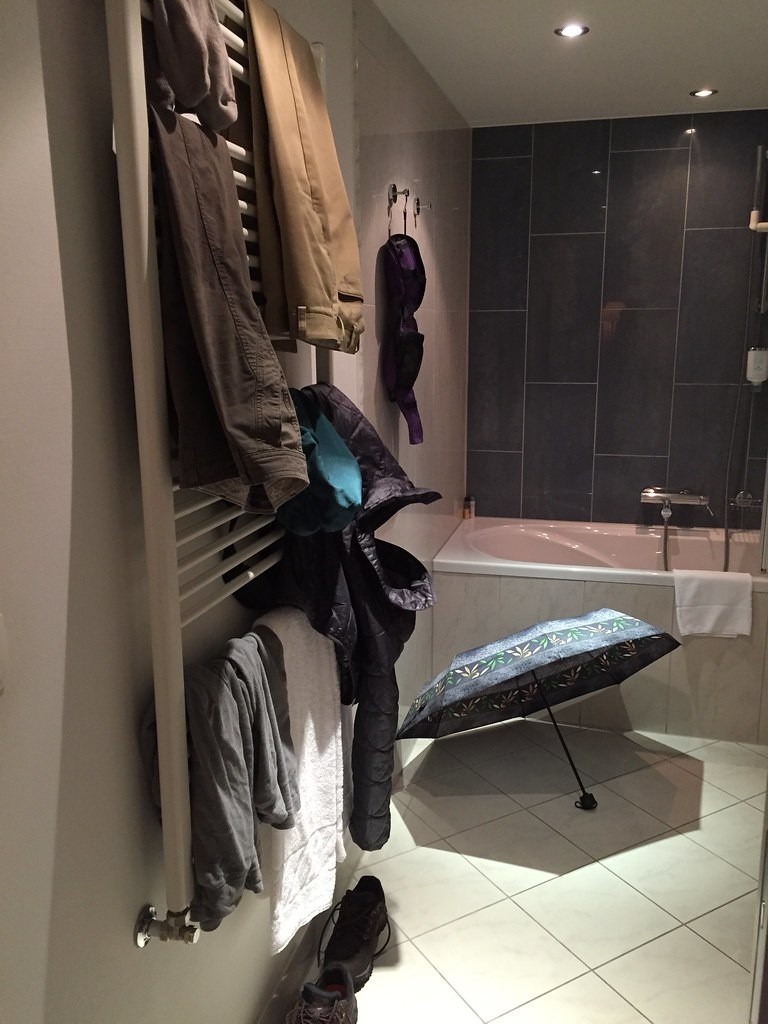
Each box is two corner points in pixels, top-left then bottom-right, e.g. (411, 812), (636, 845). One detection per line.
(432, 515), (768, 746)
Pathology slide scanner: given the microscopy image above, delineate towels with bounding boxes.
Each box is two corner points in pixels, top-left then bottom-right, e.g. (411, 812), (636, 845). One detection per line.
(254, 606), (348, 962)
(672, 569), (754, 640)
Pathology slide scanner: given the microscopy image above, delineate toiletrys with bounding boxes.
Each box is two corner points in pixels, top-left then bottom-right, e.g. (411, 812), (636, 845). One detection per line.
(463, 496), (476, 520)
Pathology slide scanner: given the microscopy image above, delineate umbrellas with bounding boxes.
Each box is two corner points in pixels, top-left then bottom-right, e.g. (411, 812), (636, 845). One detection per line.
(397, 606), (681, 812)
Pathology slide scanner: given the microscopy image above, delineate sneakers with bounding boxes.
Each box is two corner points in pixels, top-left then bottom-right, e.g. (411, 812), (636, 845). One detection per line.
(284, 962), (359, 1024)
(317, 873), (392, 992)
